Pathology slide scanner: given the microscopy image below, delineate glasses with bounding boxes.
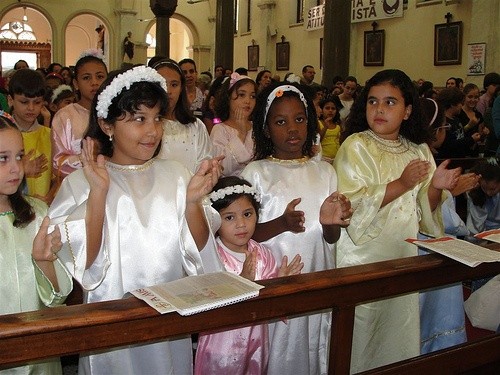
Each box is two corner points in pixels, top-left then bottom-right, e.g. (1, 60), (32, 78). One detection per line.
(307, 71), (316, 75)
(432, 123), (451, 134)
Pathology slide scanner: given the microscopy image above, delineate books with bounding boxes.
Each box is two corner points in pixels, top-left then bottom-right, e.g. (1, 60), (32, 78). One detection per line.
(474, 229), (500, 243)
(405, 236), (500, 267)
(122, 270), (265, 315)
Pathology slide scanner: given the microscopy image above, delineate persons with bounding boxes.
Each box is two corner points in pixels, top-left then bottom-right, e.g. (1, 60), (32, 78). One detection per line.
(317, 99), (342, 164)
(0, 55), (500, 375)
(51, 48), (109, 182)
(203, 72), (257, 176)
(333, 69), (462, 375)
